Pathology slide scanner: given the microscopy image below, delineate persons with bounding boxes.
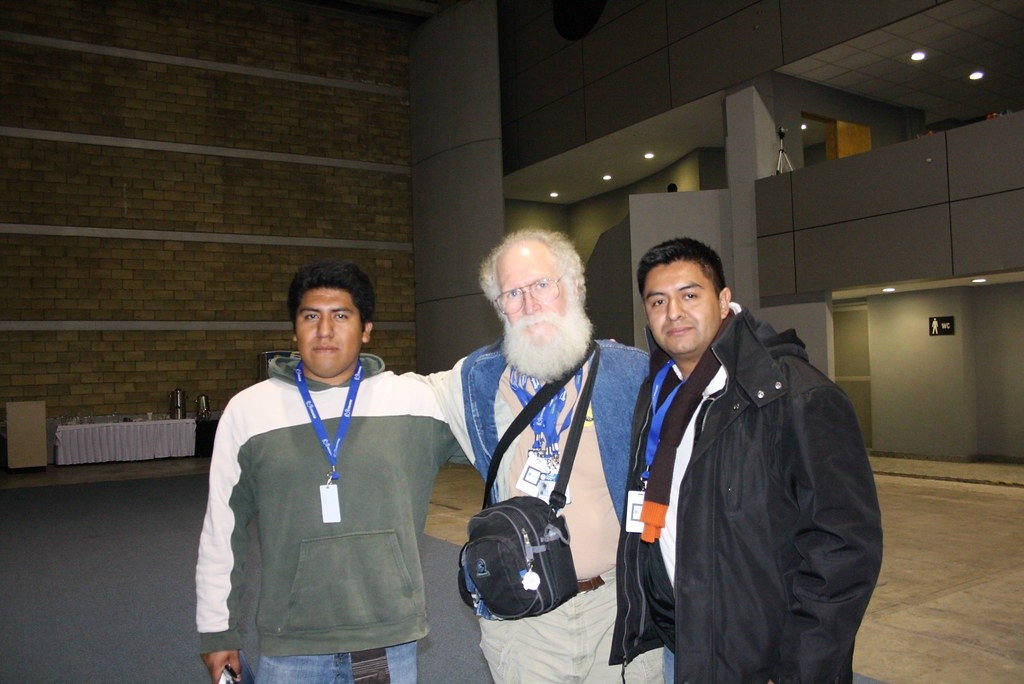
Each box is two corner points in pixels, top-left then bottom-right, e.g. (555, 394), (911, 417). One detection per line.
(399, 228), (674, 684)
(607, 236), (883, 684)
(196, 263), (459, 684)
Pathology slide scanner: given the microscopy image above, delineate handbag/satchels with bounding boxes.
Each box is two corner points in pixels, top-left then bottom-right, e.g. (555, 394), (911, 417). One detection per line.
(456, 496), (579, 620)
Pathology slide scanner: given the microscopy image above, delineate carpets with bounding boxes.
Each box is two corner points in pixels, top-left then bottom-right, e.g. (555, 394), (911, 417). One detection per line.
(0, 473), (883, 684)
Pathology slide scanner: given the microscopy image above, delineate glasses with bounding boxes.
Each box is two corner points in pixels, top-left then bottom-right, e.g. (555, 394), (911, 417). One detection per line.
(494, 273), (569, 315)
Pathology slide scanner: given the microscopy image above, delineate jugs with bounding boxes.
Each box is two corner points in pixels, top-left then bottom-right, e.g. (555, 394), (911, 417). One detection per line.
(169, 388), (187, 420)
(195, 394), (211, 416)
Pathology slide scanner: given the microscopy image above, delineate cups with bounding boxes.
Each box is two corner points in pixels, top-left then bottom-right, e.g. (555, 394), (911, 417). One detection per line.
(112, 411), (120, 423)
(147, 413), (153, 420)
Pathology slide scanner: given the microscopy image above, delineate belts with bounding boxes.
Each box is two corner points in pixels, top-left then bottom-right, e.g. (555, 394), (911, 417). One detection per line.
(577, 576), (605, 592)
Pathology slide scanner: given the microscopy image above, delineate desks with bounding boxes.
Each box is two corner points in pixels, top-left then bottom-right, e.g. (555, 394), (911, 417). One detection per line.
(54, 418), (197, 465)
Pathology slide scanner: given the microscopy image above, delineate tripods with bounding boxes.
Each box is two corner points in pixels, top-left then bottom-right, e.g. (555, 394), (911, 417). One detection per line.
(776, 126), (794, 176)
